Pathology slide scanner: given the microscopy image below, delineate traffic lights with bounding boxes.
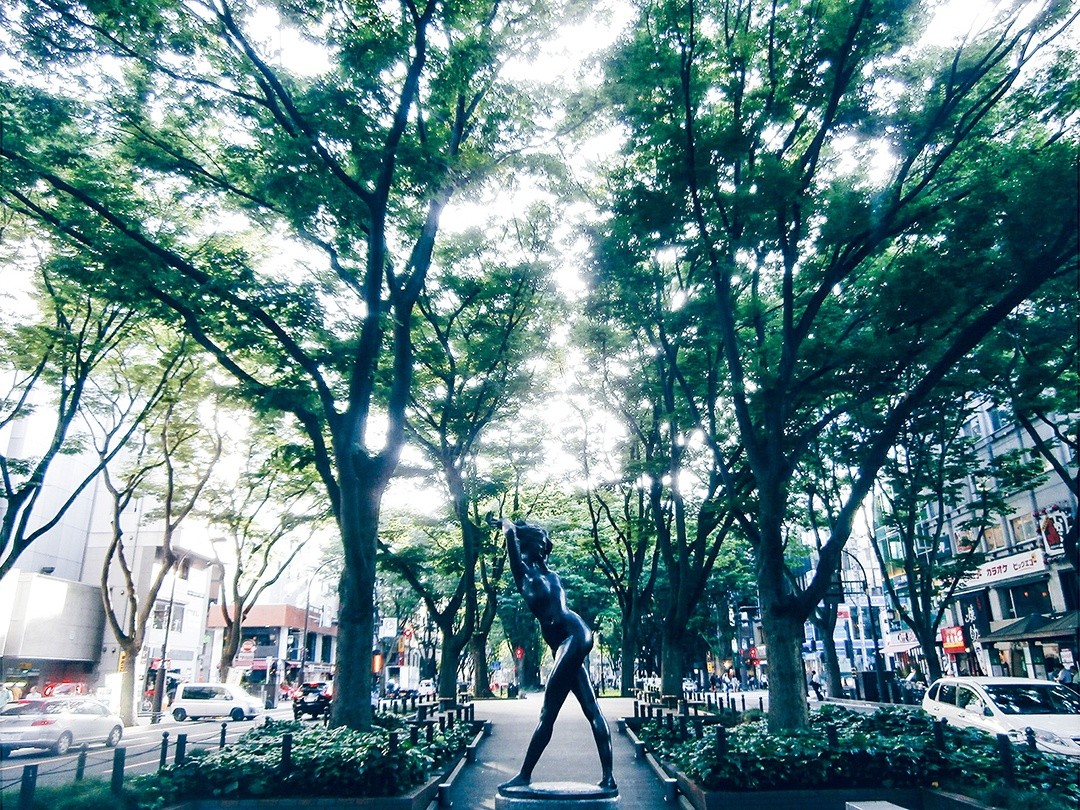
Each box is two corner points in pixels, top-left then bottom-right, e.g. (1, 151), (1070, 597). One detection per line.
(405, 629), (412, 638)
(515, 650), (523, 659)
(750, 648), (756, 659)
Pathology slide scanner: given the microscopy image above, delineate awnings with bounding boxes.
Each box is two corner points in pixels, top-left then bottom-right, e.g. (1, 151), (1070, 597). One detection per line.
(979, 612), (1080, 644)
(873, 643), (919, 654)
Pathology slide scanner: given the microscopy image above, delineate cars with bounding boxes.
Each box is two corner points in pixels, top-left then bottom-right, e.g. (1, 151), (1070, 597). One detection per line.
(920, 676), (1080, 767)
(0, 697), (125, 760)
(683, 678), (699, 693)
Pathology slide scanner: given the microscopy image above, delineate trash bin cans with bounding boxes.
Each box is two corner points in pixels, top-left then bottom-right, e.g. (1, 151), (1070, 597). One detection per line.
(460, 685), (467, 692)
(856, 670), (895, 703)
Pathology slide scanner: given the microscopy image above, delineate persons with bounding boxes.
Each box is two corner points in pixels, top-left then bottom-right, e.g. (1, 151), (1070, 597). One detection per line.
(0, 683), (41, 709)
(810, 670), (824, 701)
(693, 673), (767, 694)
(278, 681), (295, 701)
(1053, 663), (1080, 684)
(485, 512), (615, 788)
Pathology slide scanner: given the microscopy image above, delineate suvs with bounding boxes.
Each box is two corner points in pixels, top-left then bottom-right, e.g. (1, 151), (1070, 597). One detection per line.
(293, 682), (334, 720)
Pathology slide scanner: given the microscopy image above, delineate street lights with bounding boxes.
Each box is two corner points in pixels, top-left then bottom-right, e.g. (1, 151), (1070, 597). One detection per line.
(598, 632), (606, 695)
(149, 529), (226, 723)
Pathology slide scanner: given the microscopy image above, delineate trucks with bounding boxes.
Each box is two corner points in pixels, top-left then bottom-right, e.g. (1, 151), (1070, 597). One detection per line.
(385, 665), (420, 695)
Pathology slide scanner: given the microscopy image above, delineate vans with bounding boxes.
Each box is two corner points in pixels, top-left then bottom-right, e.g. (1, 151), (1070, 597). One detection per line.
(168, 682), (266, 721)
(418, 681), (433, 695)
